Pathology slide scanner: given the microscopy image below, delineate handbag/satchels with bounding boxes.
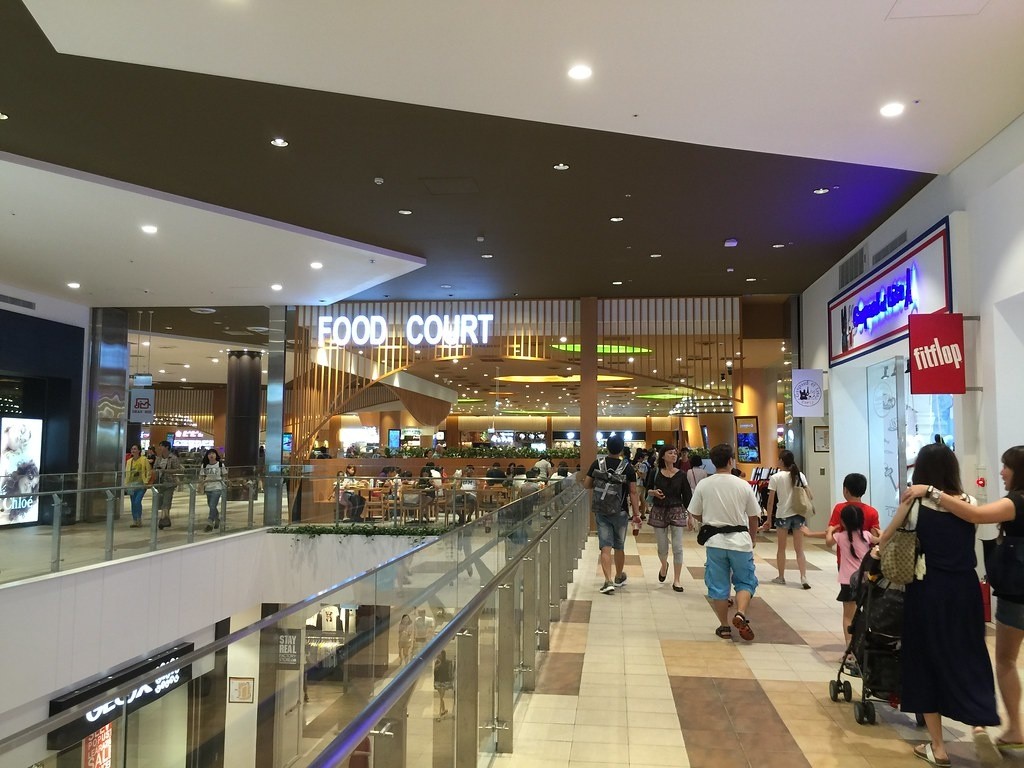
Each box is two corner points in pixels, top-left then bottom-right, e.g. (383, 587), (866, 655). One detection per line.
(880, 497), (920, 583)
(791, 476), (815, 518)
(978, 534), (1024, 603)
(647, 495), (654, 503)
(128, 483), (143, 495)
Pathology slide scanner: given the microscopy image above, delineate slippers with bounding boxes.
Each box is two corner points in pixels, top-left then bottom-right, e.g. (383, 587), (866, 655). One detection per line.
(995, 737), (1024, 749)
(974, 730), (1000, 766)
(913, 742), (951, 767)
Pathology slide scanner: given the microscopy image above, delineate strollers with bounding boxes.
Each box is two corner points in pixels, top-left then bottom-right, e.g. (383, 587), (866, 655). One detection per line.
(827, 541), (927, 728)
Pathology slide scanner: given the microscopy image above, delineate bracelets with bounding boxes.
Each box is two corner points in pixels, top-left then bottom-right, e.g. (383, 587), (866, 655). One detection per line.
(876, 548), (881, 557)
(926, 485), (943, 504)
(634, 515), (639, 516)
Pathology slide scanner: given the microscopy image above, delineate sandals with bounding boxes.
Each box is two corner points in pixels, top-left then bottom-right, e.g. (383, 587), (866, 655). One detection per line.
(727, 598), (733, 607)
(732, 612), (754, 641)
(715, 626), (732, 639)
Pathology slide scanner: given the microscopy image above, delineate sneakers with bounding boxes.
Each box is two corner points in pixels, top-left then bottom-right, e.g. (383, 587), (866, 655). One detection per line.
(614, 572), (627, 587)
(772, 577), (786, 583)
(600, 581), (615, 593)
(801, 576), (812, 589)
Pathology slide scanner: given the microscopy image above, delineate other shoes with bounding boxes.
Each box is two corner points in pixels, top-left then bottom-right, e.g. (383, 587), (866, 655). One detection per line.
(129, 517), (220, 532)
(458, 515), (463, 523)
(628, 506), (651, 521)
(659, 562), (669, 582)
(838, 652), (855, 664)
(673, 583), (683, 592)
(467, 516), (471, 522)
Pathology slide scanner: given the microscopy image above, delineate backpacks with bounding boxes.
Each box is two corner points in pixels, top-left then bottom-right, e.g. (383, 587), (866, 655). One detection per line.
(592, 457), (629, 516)
(637, 462), (653, 489)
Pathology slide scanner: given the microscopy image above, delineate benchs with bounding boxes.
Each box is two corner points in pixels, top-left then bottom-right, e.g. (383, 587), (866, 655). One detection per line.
(309, 479), (376, 521)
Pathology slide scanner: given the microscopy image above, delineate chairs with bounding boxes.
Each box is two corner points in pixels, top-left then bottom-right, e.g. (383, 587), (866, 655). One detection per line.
(364, 482), (513, 528)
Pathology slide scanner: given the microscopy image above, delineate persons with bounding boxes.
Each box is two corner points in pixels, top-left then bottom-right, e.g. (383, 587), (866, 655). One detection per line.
(397, 609), (434, 667)
(622, 447), (707, 532)
(317, 446), (331, 459)
(583, 435), (642, 593)
(825, 504), (882, 663)
(870, 442), (1005, 768)
(0, 426), (40, 521)
(800, 473), (880, 574)
(763, 450), (813, 589)
(433, 650), (453, 716)
(686, 444), (761, 641)
(485, 454), (580, 524)
(371, 462), (480, 523)
(125, 440), (185, 530)
(424, 444), (443, 458)
(646, 445), (695, 592)
(197, 448), (228, 532)
(328, 464), (368, 522)
(900, 446), (1024, 750)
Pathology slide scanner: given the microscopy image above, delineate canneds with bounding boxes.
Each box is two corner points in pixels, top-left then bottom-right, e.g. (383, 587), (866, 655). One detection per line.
(632, 523), (639, 536)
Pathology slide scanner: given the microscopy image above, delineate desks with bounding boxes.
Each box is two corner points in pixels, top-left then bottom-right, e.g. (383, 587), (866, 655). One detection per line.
(353, 487), (385, 522)
(407, 488), (437, 525)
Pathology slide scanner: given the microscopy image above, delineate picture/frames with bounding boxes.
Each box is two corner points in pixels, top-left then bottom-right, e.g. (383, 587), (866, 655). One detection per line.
(229, 677), (254, 703)
(813, 425), (829, 452)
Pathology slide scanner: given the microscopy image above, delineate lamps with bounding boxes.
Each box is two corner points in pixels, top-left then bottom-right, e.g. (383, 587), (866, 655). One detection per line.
(720, 374), (726, 382)
